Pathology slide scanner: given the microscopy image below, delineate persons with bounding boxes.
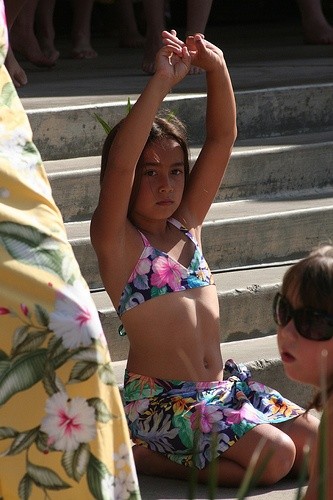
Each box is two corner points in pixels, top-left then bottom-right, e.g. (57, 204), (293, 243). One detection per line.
(89, 28), (321, 487)
(0, 1), (333, 89)
(271, 244), (333, 500)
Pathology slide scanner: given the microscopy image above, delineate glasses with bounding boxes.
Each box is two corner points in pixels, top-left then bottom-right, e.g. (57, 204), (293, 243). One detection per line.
(271, 293), (333, 340)
